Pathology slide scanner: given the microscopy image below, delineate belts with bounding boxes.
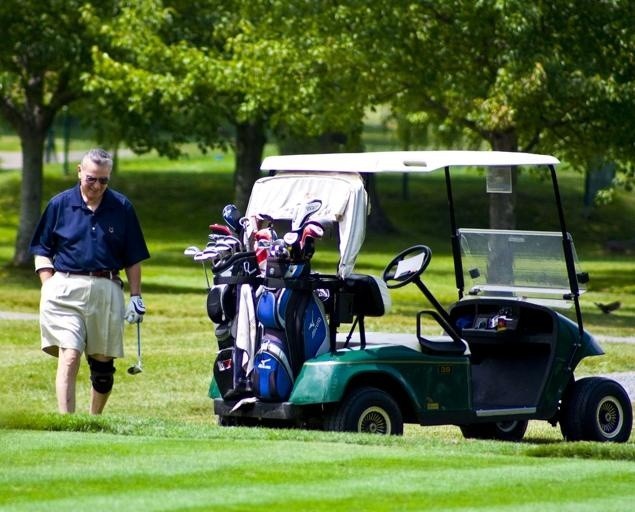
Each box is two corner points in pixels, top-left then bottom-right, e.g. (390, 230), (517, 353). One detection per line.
(73, 271), (124, 288)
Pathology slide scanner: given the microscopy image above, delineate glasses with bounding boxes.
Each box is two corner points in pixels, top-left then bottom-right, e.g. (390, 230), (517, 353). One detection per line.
(85, 175), (109, 184)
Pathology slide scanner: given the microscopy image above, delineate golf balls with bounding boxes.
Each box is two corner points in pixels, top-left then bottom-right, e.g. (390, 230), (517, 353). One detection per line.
(127, 315), (134, 322)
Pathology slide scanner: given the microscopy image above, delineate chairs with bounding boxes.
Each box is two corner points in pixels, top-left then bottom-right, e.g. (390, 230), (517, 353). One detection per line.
(321, 271), (472, 357)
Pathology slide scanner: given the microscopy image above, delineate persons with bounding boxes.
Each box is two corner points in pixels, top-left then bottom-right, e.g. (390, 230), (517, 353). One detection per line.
(28, 148), (150, 415)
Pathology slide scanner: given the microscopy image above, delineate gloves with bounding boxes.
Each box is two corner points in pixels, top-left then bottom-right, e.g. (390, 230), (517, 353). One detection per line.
(123, 295), (145, 323)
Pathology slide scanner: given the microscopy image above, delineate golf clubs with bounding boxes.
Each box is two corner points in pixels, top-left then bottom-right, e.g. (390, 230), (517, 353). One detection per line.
(184, 200), (325, 289)
(128, 320), (142, 374)
